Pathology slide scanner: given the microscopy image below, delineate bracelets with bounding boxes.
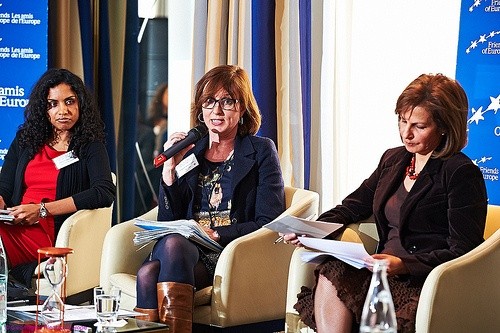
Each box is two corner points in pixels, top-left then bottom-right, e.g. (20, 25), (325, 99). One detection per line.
(213, 231), (219, 237)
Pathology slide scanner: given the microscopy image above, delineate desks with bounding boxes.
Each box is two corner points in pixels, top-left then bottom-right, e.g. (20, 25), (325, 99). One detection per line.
(7, 298), (169, 333)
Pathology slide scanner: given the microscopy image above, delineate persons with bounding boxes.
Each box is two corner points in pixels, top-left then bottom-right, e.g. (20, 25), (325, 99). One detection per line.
(134, 66), (285, 333)
(133, 86), (168, 202)
(284, 74), (488, 333)
(0, 69), (116, 269)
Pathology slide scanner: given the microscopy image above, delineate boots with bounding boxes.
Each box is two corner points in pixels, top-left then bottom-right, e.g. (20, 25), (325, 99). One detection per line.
(157, 281), (193, 333)
(135, 306), (158, 322)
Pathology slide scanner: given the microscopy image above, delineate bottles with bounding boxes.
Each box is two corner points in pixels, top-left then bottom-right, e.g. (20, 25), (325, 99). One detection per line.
(359, 258), (398, 333)
(0, 236), (9, 324)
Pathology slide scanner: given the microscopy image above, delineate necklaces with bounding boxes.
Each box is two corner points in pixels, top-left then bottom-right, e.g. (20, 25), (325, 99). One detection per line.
(406, 154), (418, 180)
(203, 152), (234, 228)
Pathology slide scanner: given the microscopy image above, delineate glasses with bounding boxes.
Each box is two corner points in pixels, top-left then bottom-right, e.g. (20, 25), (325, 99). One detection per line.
(201, 97), (242, 110)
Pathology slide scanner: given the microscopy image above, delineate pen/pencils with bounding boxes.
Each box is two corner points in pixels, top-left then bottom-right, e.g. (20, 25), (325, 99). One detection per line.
(274, 237), (283, 245)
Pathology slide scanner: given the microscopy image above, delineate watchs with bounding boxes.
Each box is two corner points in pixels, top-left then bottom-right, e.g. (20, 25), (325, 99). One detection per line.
(39, 203), (47, 218)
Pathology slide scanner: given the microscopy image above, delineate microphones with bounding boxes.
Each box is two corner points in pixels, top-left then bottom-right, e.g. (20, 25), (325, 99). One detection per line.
(153, 125), (209, 168)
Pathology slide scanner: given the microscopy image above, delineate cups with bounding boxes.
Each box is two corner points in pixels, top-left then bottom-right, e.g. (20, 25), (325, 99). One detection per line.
(93, 286), (122, 319)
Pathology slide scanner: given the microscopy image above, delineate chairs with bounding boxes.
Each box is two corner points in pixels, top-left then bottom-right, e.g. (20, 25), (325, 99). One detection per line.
(99, 186), (320, 333)
(285, 205), (500, 333)
(30, 171), (117, 306)
